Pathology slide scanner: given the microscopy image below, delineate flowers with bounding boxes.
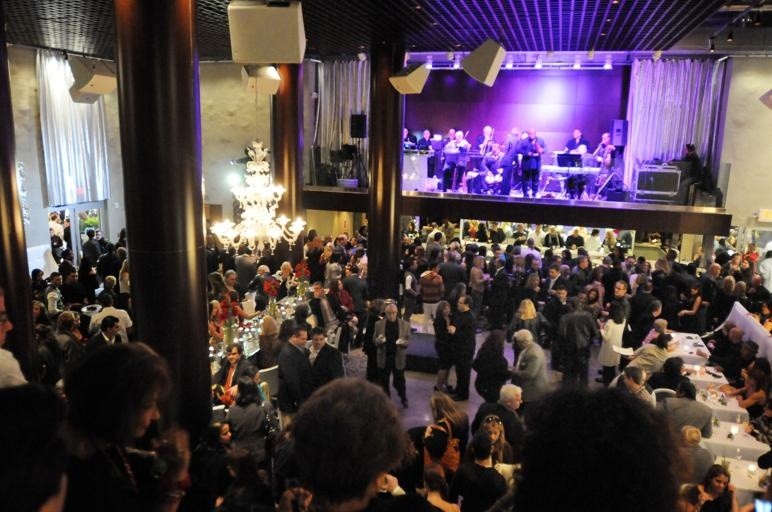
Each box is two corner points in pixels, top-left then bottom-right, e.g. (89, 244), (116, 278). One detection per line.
(292, 261), (311, 298)
(215, 300), (241, 325)
(260, 275), (280, 309)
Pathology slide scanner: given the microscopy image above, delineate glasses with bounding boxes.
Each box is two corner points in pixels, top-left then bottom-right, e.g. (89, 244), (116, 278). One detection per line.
(484, 416), (502, 426)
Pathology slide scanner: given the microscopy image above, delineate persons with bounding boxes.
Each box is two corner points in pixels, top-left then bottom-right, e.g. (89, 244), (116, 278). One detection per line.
(674, 143), (703, 205)
(402, 126), (544, 199)
(591, 132), (617, 189)
(1, 210), (772, 512)
(562, 131), (589, 156)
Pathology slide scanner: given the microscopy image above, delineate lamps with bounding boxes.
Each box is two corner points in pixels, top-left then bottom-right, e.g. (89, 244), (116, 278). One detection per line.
(208, 65), (306, 257)
(422, 47), (663, 70)
(709, 1), (761, 53)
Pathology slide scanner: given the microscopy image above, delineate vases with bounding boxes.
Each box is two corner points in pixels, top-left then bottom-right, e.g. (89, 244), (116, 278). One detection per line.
(268, 295), (278, 320)
(297, 280), (306, 301)
(222, 321), (234, 348)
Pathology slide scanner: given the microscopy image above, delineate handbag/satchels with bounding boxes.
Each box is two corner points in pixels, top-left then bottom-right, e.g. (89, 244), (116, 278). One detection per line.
(264, 427), (278, 458)
(424, 437), (461, 473)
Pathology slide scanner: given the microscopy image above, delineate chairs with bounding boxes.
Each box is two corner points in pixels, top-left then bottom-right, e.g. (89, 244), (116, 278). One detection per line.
(212, 404), (226, 425)
(257, 363), (280, 402)
(329, 327), (347, 378)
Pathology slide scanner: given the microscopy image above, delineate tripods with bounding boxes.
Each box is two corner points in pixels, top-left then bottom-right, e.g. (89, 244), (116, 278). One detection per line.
(347, 139), (367, 187)
(592, 149), (629, 201)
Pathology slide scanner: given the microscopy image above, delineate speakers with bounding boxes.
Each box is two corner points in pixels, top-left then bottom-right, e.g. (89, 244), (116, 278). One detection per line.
(611, 118), (627, 146)
(341, 143), (356, 160)
(349, 114), (366, 138)
(330, 150), (343, 163)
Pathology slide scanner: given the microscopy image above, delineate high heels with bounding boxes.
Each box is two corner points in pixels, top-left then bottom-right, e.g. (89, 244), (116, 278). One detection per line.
(432, 381), (454, 391)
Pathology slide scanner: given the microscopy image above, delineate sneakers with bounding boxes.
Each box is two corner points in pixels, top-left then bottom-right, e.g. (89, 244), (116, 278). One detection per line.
(595, 376), (606, 384)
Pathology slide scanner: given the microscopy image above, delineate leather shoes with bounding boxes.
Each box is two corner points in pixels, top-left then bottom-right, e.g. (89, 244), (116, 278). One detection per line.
(450, 388), (470, 401)
